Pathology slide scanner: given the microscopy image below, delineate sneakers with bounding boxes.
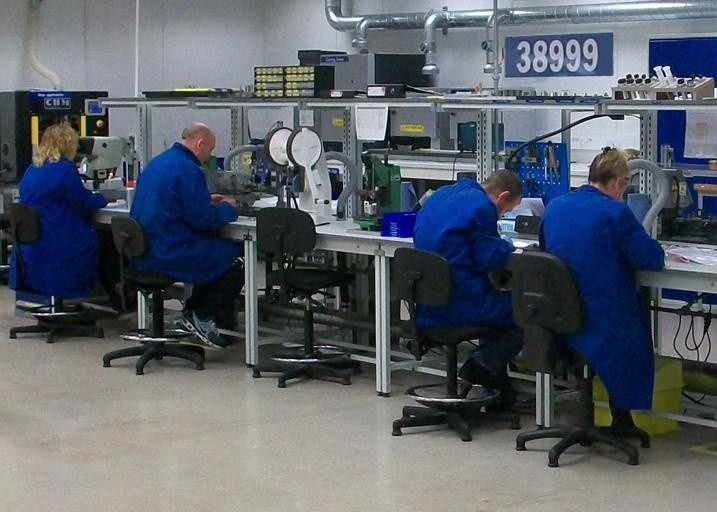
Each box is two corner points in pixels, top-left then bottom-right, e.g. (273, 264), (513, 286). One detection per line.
(178, 311), (229, 349)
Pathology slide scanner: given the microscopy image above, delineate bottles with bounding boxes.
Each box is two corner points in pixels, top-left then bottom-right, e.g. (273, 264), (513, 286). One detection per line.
(616, 65), (705, 98)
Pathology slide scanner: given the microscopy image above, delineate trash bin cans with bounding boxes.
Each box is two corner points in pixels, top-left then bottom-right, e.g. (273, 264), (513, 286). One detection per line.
(592, 354), (685, 436)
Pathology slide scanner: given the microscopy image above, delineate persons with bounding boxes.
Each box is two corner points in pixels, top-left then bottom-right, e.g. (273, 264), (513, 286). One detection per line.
(537, 150), (666, 447)
(412, 168), (525, 404)
(127, 122), (244, 351)
(9, 123), (135, 312)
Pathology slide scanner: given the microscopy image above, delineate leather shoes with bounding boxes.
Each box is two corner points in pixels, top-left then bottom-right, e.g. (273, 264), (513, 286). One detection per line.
(460, 360), (516, 397)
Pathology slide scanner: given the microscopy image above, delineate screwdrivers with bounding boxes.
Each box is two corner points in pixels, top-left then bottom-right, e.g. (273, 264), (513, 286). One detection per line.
(543, 147), (549, 182)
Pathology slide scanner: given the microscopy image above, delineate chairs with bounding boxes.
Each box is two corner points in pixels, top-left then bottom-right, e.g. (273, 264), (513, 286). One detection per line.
(10, 203), (105, 344)
(388, 246), (523, 440)
(507, 247), (651, 467)
